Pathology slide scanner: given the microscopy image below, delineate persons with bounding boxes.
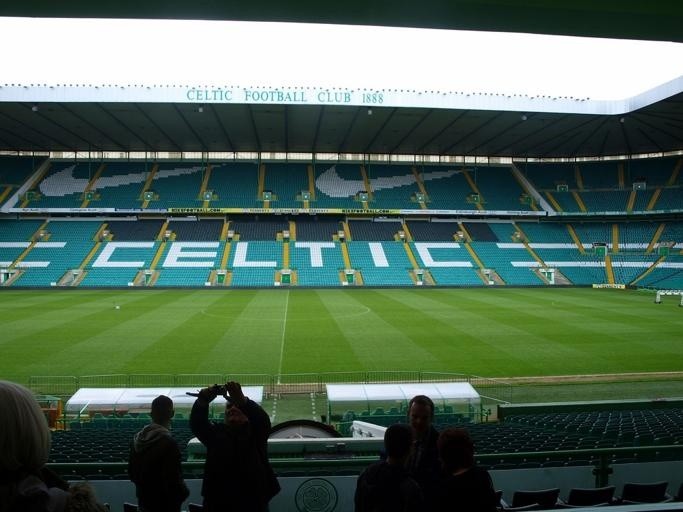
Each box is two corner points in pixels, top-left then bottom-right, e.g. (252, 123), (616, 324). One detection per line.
(127, 394), (191, 510)
(353, 423), (426, 511)
(426, 421), (500, 510)
(43, 472), (71, 495)
(0, 378), (104, 509)
(188, 380), (281, 511)
(67, 483), (110, 511)
(592, 455), (614, 487)
(379, 393), (441, 476)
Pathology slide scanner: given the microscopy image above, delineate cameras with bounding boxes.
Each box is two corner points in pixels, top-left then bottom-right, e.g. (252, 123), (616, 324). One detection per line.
(212, 384), (227, 395)
